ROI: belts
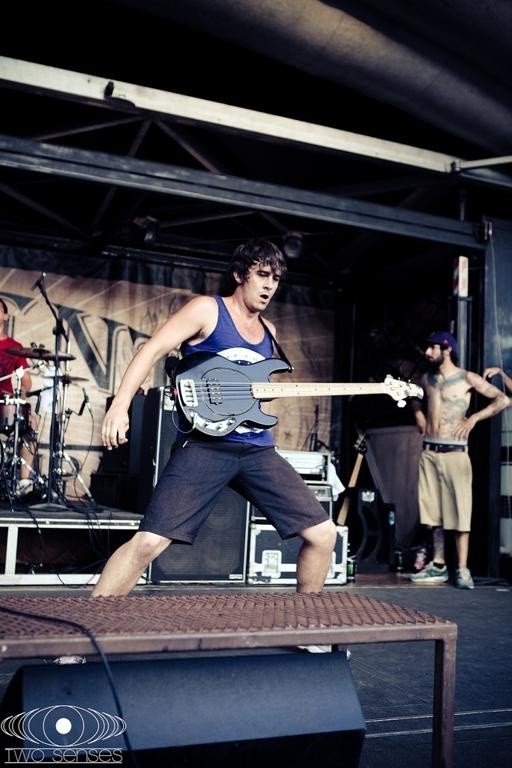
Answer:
[421,440,463,452]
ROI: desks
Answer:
[1,591,458,767]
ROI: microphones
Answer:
[31,272,47,292]
[26,387,52,397]
[310,405,318,451]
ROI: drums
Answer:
[0,397,32,436]
[0,440,20,501]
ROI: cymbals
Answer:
[44,375,89,382]
[4,347,77,361]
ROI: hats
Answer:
[415,330,459,356]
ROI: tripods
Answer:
[9,285,85,514]
[0,404,53,503]
[43,382,97,507]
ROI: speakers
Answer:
[0,652,366,768]
[142,387,251,586]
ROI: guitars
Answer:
[173,351,425,438]
[333,432,370,557]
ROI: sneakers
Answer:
[455,567,475,589]
[409,560,448,583]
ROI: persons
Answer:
[0,299,38,499]
[54,237,351,664]
[482,367,512,393]
[409,330,510,590]
